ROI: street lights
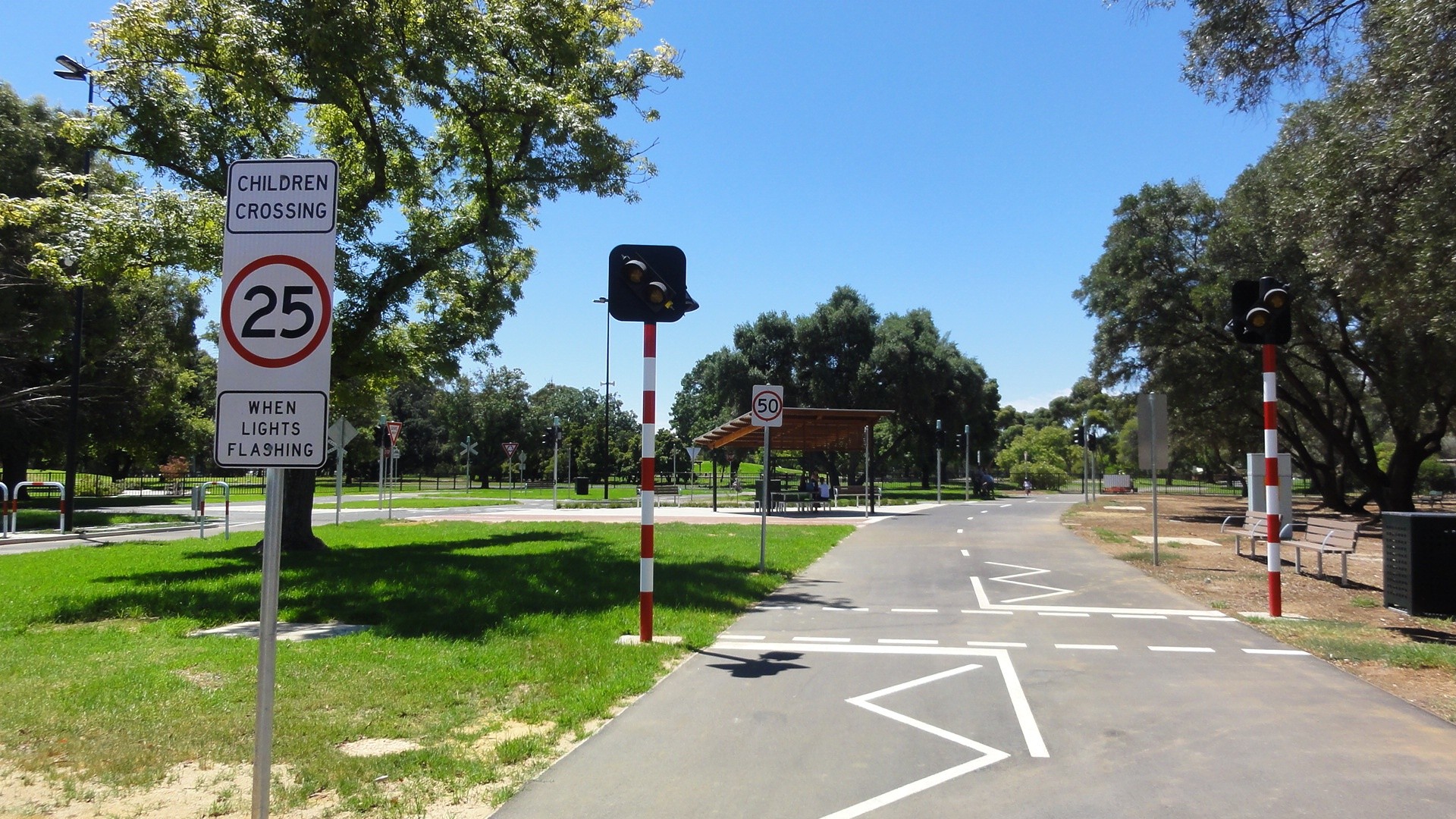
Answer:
[52,53,116,532]
[592,297,609,499]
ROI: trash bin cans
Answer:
[1382,511,1456,620]
[754,480,782,508]
[573,477,589,495]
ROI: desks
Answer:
[770,492,820,513]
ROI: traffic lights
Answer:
[955,432,966,456]
[372,424,383,448]
[608,244,687,323]
[1073,424,1085,447]
[1232,278,1292,345]
[542,425,555,448]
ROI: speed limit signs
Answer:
[751,384,783,427]
[220,254,331,367]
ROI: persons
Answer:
[761,474,764,479]
[1118,470,1126,475]
[971,463,996,501]
[733,479,742,492]
[1024,477,1031,495]
[798,467,829,511]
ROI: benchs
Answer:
[1221,511,1293,561]
[754,499,809,513]
[637,486,682,507]
[833,486,881,508]
[779,501,831,514]
[1417,490,1444,509]
[1280,518,1359,585]
[525,480,556,495]
